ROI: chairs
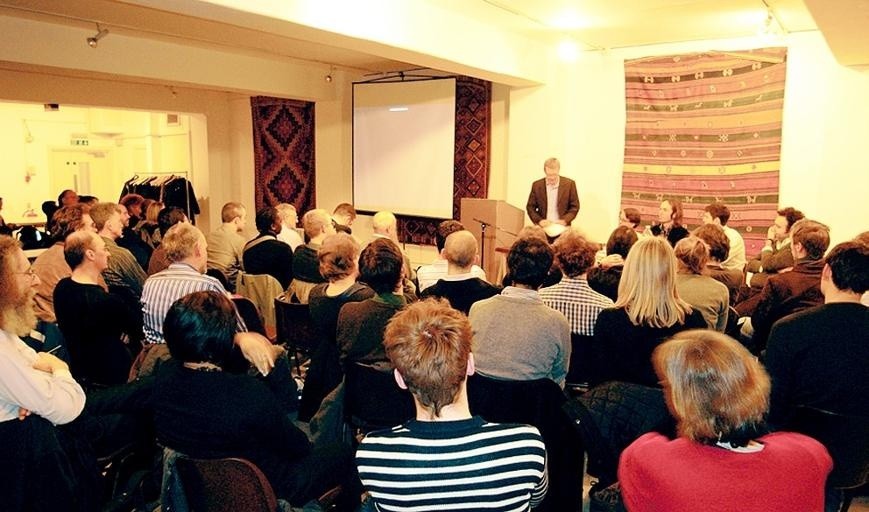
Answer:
[1,266,869,511]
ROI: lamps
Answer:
[84,19,109,48]
[322,64,339,86]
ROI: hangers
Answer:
[127,173,183,185]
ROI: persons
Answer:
[418,198,869,410]
[354,297,549,512]
[617,330,832,511]
[526,159,580,240]
[0,190,422,511]
